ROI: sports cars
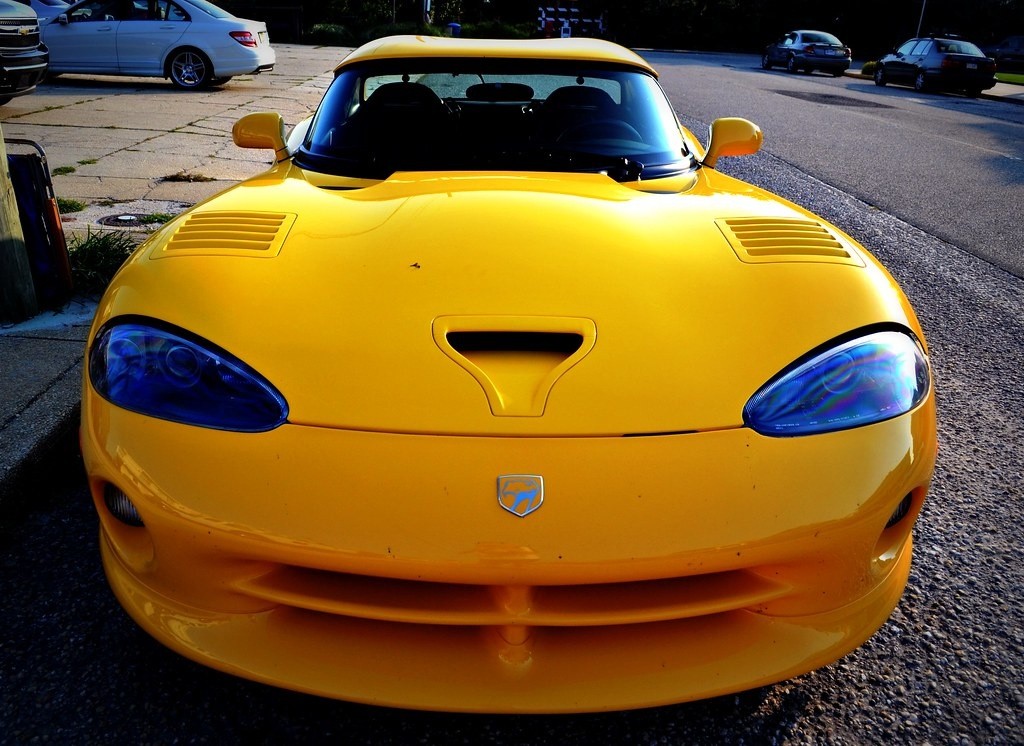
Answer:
[76,36,944,719]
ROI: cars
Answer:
[872,36,997,100]
[37,0,277,90]
[0,1,49,107]
[760,30,854,76]
[15,0,90,16]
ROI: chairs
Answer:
[155,7,166,20]
[346,80,639,172]
